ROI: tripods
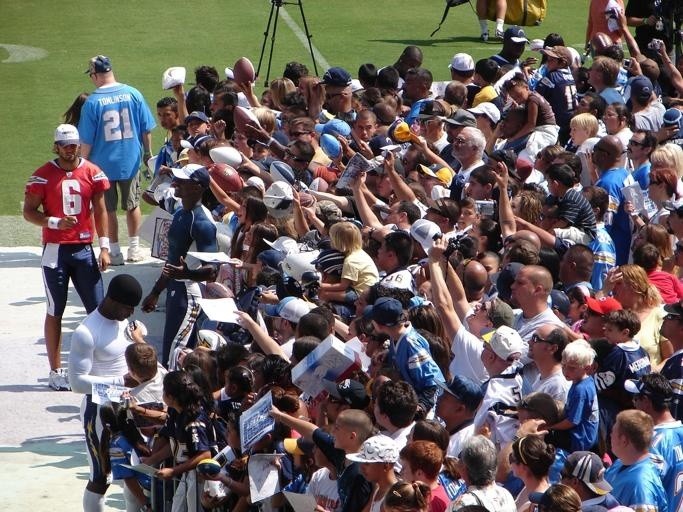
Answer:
[255,0,319,86]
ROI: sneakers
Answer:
[107,252,126,267]
[127,247,148,262]
[48,369,71,392]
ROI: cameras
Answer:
[120,395,130,408]
[623,58,633,68]
[475,200,494,216]
[648,39,663,49]
[433,232,458,256]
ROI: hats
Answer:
[108,274,142,307]
[180,134,214,151]
[262,24,683,272]
[283,435,316,459]
[363,296,403,326]
[183,110,210,127]
[258,296,310,323]
[170,164,210,188]
[54,124,82,149]
[623,379,652,396]
[564,451,615,495]
[319,377,369,410]
[479,325,524,363]
[582,295,623,318]
[83,54,112,74]
[431,375,483,406]
[344,434,401,464]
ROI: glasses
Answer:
[509,452,517,464]
[531,333,552,346]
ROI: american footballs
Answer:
[234,57,256,88]
[297,191,313,208]
[208,164,243,192]
[270,161,295,186]
[309,177,329,193]
[320,133,340,158]
[514,158,535,182]
[281,396,310,420]
[205,281,235,298]
[590,32,613,56]
[210,147,242,169]
[233,105,260,137]
[196,459,222,476]
[161,66,186,89]
